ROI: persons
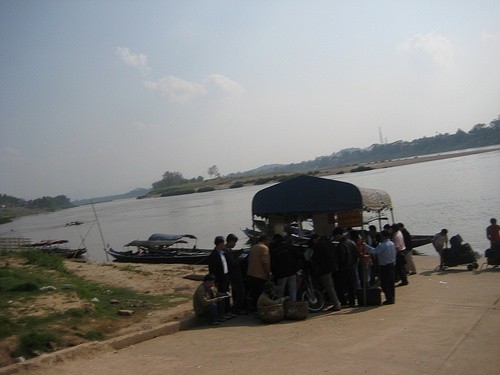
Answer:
[486,218,500,268]
[193,223,417,326]
[432,229,448,271]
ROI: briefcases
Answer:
[356,285,381,306]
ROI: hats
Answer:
[389,224,400,229]
[264,281,277,291]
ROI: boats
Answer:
[242,213,437,250]
[20,239,87,258]
[108,232,243,265]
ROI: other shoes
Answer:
[383,300,394,305]
[209,321,219,324]
[219,316,230,319]
[398,282,408,286]
[227,312,237,317]
[216,318,226,322]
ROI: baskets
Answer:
[284,301,308,320]
[269,304,285,322]
